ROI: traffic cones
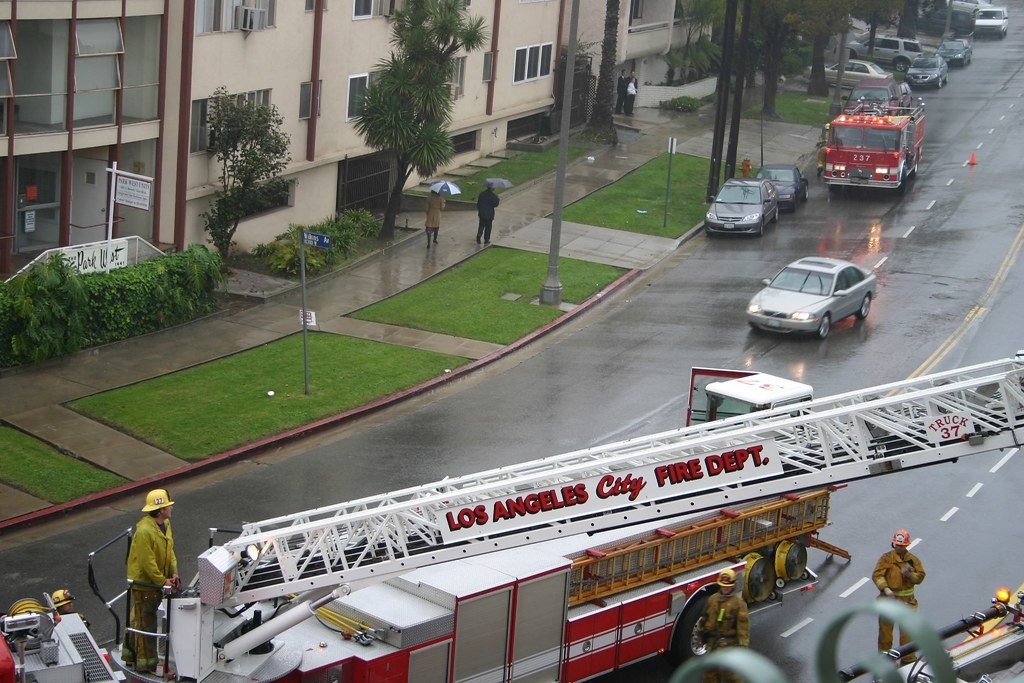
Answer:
[967,152,978,165]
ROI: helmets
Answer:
[51,590,74,607]
[892,529,910,546]
[717,569,736,587]
[141,486,175,512]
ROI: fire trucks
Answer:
[0,350,1024,683]
[822,97,925,195]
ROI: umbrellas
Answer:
[480,178,514,188]
[427,180,462,195]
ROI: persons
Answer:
[624,77,638,116]
[872,529,926,655]
[615,69,629,114]
[52,589,89,628]
[121,489,180,674]
[626,71,638,114]
[691,569,751,683]
[424,190,445,248]
[476,187,500,244]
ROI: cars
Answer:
[756,163,809,213]
[704,177,779,238]
[946,0,995,15]
[903,53,949,89]
[804,59,893,88]
[897,80,913,113]
[935,38,973,68]
[746,257,877,341]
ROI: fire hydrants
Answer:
[739,159,753,178]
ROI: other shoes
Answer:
[149,669,157,676]
[127,661,135,666]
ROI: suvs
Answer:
[841,78,902,117]
[847,34,923,72]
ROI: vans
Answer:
[919,7,975,36]
[972,7,1009,39]
